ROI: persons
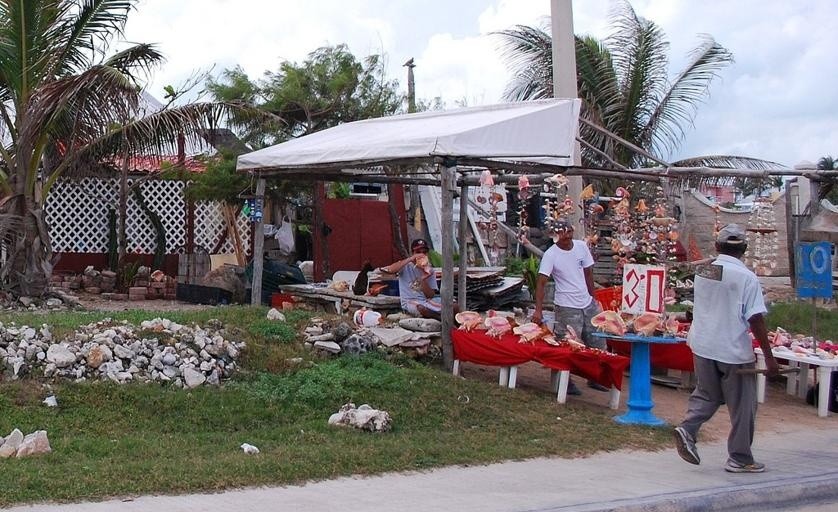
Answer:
[382,238,461,325]
[675,225,778,473]
[531,222,610,396]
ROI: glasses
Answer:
[416,251,427,253]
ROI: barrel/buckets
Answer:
[272,292,294,309]
[354,310,385,328]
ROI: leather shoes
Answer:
[587,380,609,392]
[567,375,581,395]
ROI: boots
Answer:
[673,426,700,465]
[724,456,766,473]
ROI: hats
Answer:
[411,239,430,250]
[718,223,746,244]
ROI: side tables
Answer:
[593,333,689,424]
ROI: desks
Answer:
[453,330,628,411]
[604,321,838,418]
